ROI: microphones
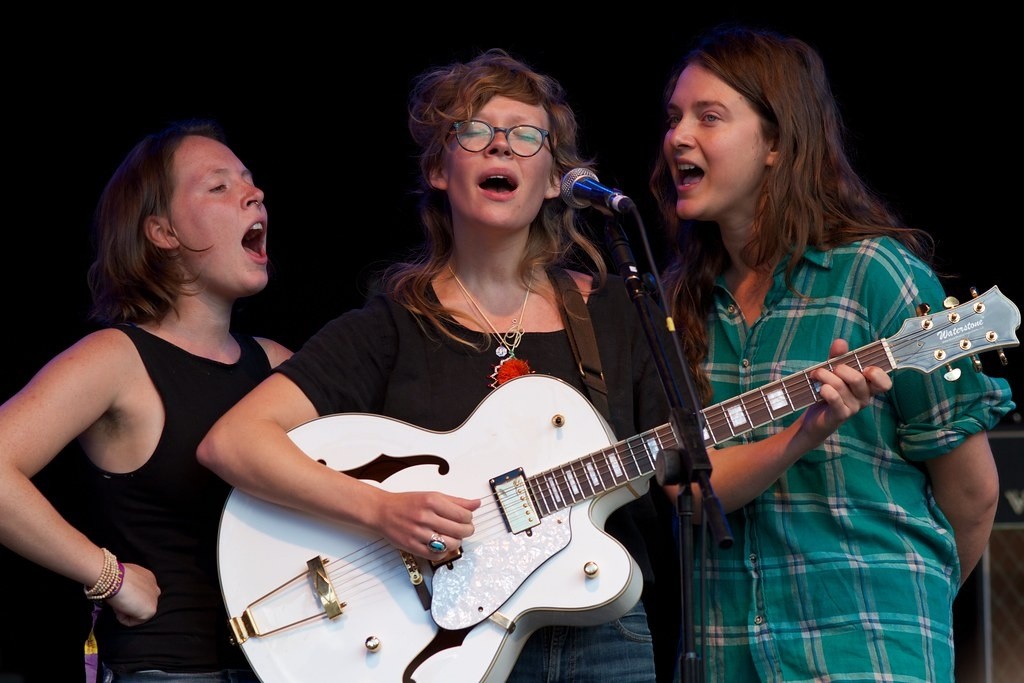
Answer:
[561,167,635,213]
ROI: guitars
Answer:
[219,285,1021,679]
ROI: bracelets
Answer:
[84,547,124,600]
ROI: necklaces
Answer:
[442,251,537,391]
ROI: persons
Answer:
[636,31,1014,683]
[0,120,301,683]
[196,52,892,683]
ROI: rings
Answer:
[427,533,447,553]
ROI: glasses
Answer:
[441,120,563,169]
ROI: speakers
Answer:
[950,430,1024,683]
